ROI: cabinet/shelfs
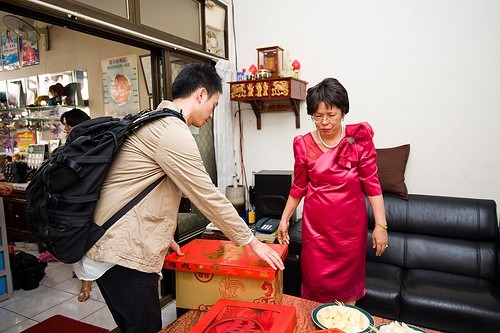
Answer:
[0,101,79,154]
[0,181,38,243]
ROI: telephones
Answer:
[255,217,281,234]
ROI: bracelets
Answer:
[376,223,388,230]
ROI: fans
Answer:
[2,14,49,52]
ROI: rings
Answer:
[385,245,388,248]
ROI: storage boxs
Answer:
[162,240,297,333]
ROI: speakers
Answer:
[255,169,297,226]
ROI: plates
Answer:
[369,323,427,333]
[311,302,374,333]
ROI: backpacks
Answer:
[26,109,186,264]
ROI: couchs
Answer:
[283,194,500,333]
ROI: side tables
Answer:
[253,168,295,225]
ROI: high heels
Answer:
[78,280,92,302]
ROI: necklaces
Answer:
[318,127,344,149]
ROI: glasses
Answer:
[311,114,341,123]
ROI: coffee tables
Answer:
[157,293,442,333]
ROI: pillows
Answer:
[376,143,410,200]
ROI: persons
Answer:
[71,63,285,333]
[5,30,14,48]
[60,109,92,302]
[0,182,14,194]
[5,155,12,164]
[277,78,388,308]
[48,83,70,105]
